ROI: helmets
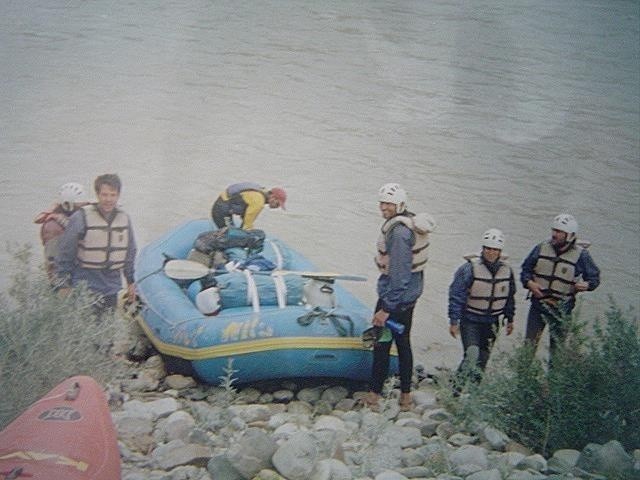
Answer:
[117,290,147,324]
[56,182,87,211]
[552,214,578,243]
[377,182,409,215]
[481,229,505,252]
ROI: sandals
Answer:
[353,392,380,405]
[398,398,412,411]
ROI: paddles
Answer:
[165,259,366,280]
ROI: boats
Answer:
[1,373,121,476]
[132,214,400,386]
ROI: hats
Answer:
[271,187,287,211]
[362,326,392,348]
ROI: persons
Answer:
[40,182,88,291]
[520,214,600,373]
[212,181,286,230]
[53,174,137,356]
[353,182,429,412]
[448,228,515,398]
[187,229,265,270]
